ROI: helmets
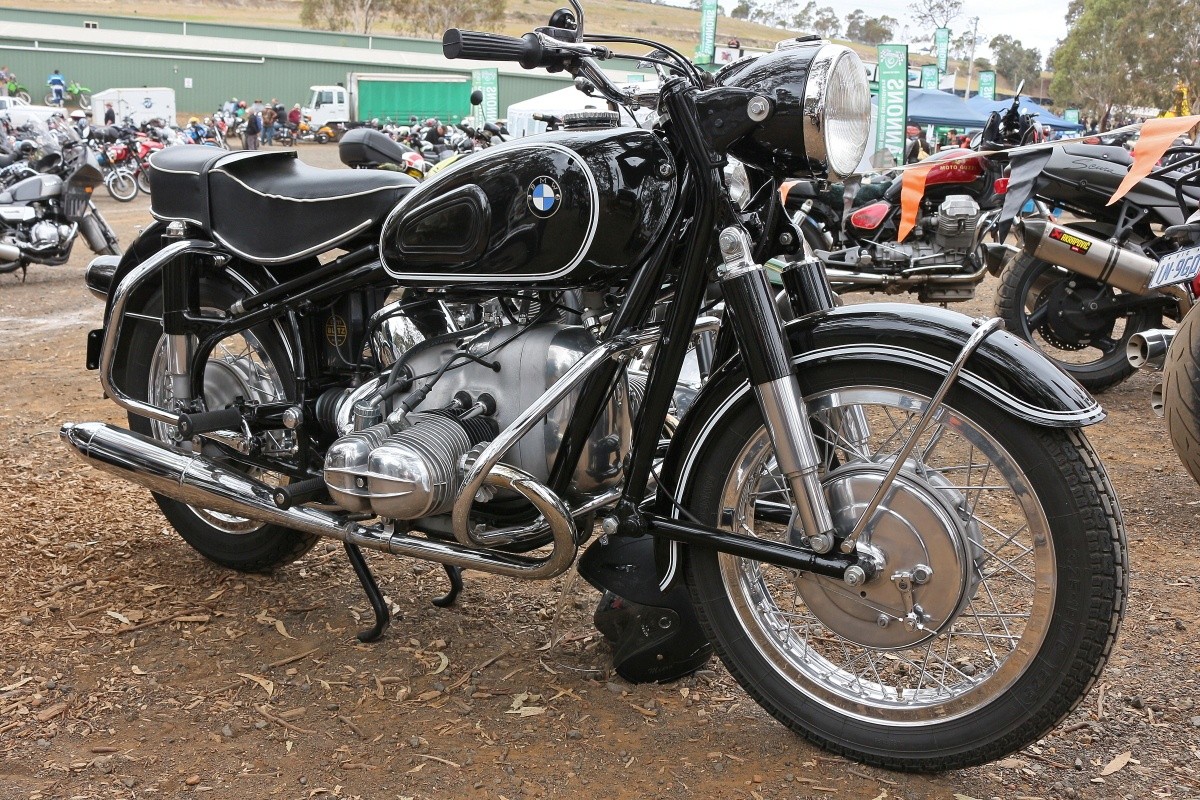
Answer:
[576,530,714,685]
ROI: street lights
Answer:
[964,16,979,101]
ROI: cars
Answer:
[0,96,68,127]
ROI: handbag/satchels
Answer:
[263,110,272,123]
[255,115,262,131]
[917,139,930,160]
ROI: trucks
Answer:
[295,70,474,132]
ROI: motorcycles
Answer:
[4,74,33,104]
[0,87,1200,492]
[43,77,92,110]
[56,0,1131,775]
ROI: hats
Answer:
[265,104,272,108]
[495,122,504,125]
[294,103,301,109]
[441,124,448,131]
[190,117,197,122]
[239,101,246,107]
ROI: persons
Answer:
[0,67,10,96]
[288,104,302,124]
[105,102,115,125]
[230,97,287,150]
[496,122,509,135]
[947,130,961,148]
[906,122,932,164]
[1080,113,1125,137]
[425,125,449,144]
[47,69,65,108]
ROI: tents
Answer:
[1106,103,1162,117]
[508,84,653,139]
[872,85,1084,154]
